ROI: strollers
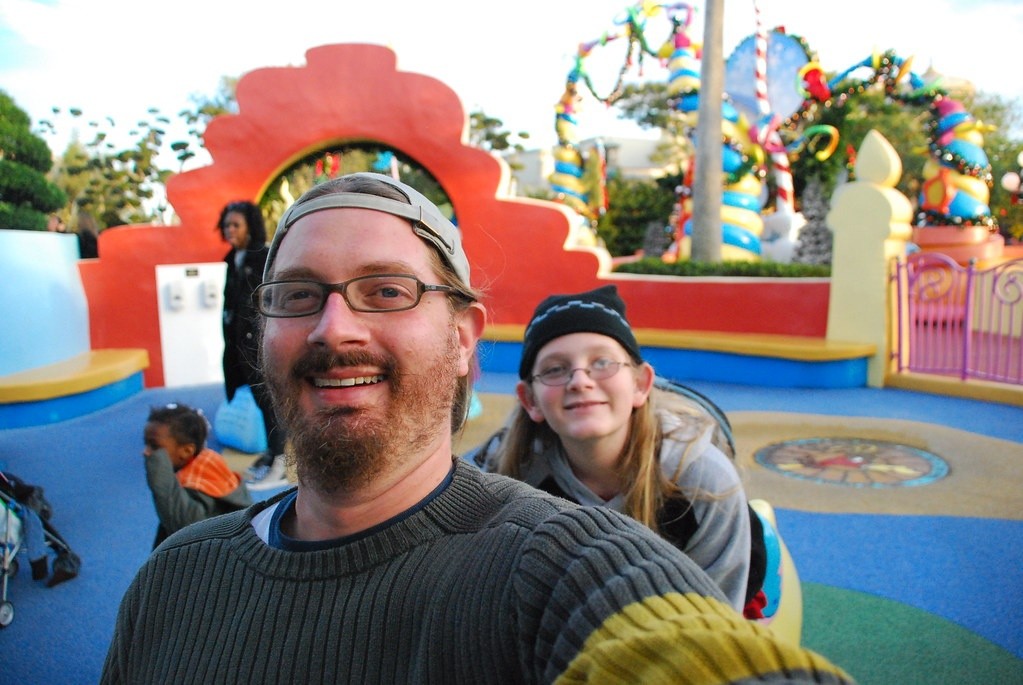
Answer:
[1,469,82,626]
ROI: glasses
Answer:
[528,355,631,385]
[252,274,454,319]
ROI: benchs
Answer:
[1,350,149,429]
[474,323,876,390]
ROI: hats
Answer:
[256,171,469,291]
[518,284,642,379]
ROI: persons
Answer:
[216,201,293,489]
[101,170,865,684]
[142,401,255,555]
[476,285,755,624]
